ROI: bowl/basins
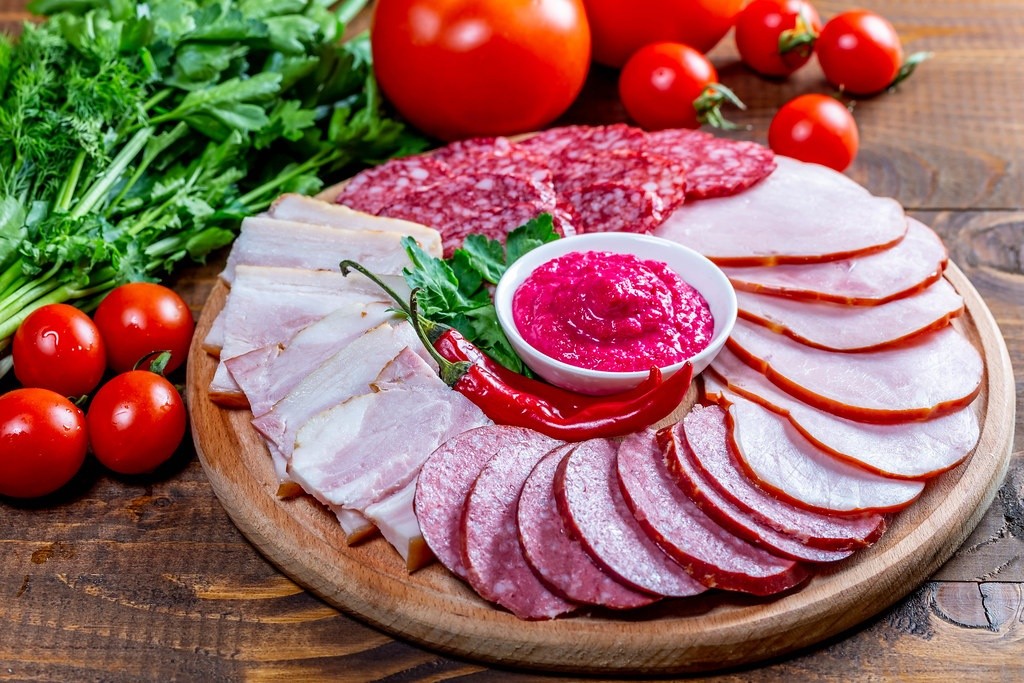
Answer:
[494,231,738,394]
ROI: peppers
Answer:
[339,260,692,442]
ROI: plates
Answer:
[186,131,1017,669]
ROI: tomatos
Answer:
[0,281,192,497]
[368,0,933,174]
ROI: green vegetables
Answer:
[0,0,427,349]
[395,212,566,378]
